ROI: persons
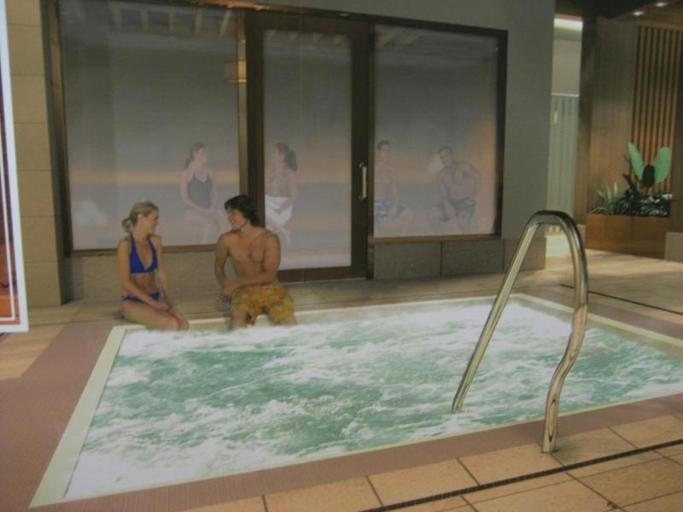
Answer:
[116,199,190,331]
[264,142,300,249]
[426,145,481,233]
[213,194,298,331]
[181,142,220,242]
[374,139,413,237]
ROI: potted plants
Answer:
[583,181,671,257]
